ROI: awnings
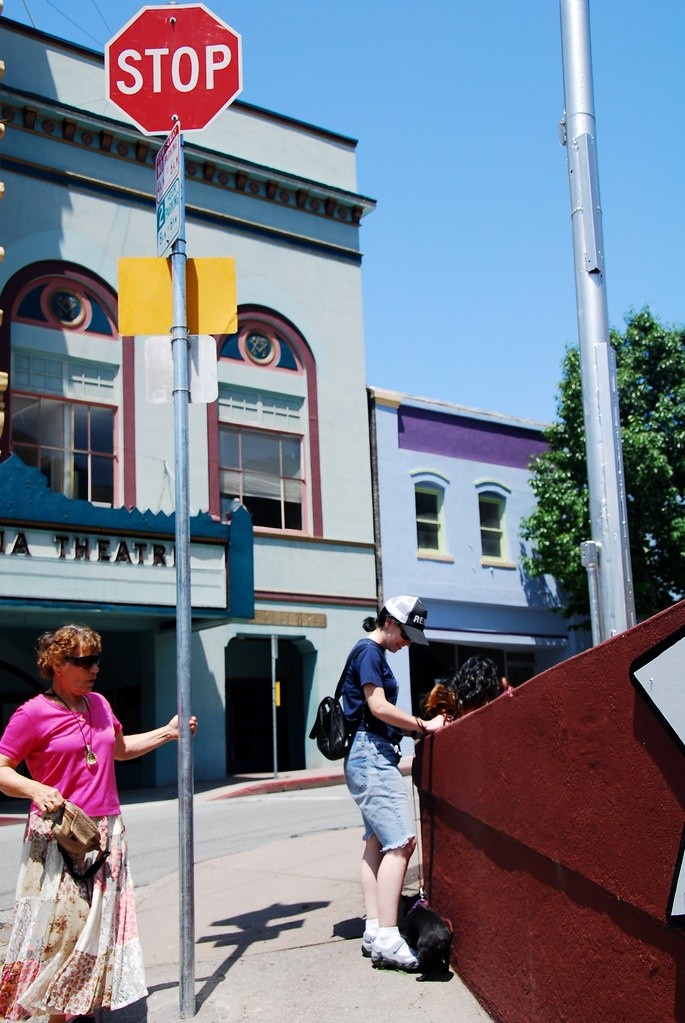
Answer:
[422,629,568,653]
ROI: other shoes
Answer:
[361,931,376,957]
[371,938,420,973]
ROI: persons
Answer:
[420,656,513,724]
[343,597,445,971]
[0,623,196,1023]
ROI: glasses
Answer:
[65,655,102,669]
[396,624,411,640]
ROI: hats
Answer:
[384,595,431,646]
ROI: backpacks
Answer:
[309,642,379,761]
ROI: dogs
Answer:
[397,891,454,982]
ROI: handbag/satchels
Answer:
[50,799,112,881]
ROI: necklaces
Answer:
[51,686,97,765]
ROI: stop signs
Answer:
[105,3,244,136]
